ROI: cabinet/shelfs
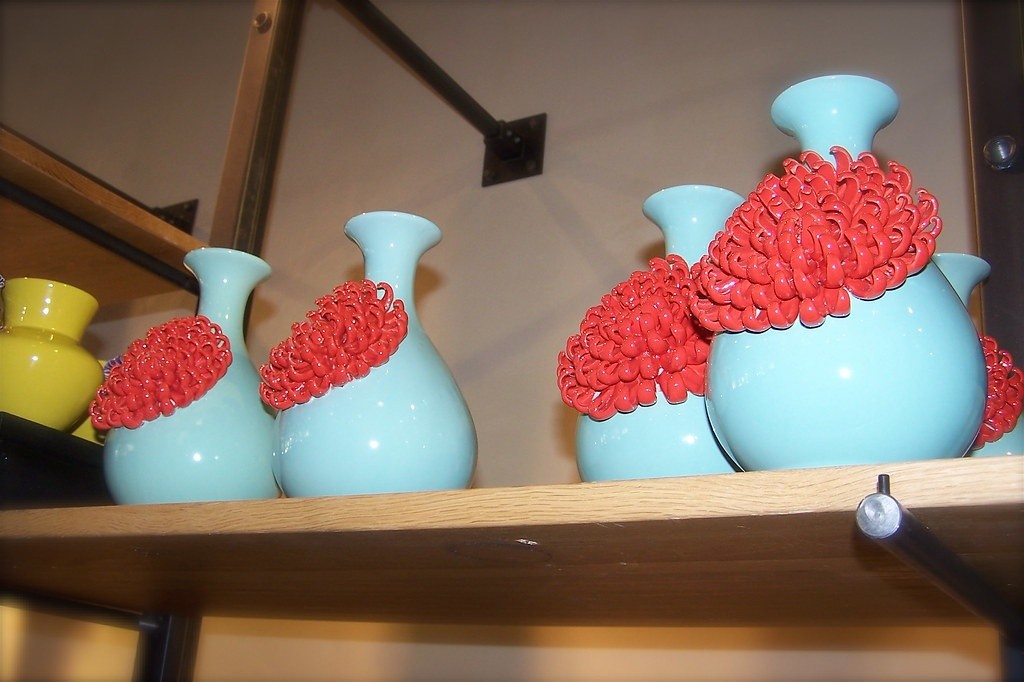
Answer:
[1,0,1024,682]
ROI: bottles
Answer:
[558,184,746,482]
[0,277,102,432]
[690,76,986,470]
[930,253,1023,460]
[260,210,476,496]
[93,245,278,498]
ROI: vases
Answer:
[268,211,479,496]
[0,276,105,430]
[928,250,1024,458]
[704,73,988,471]
[576,185,736,480]
[104,247,282,505]
